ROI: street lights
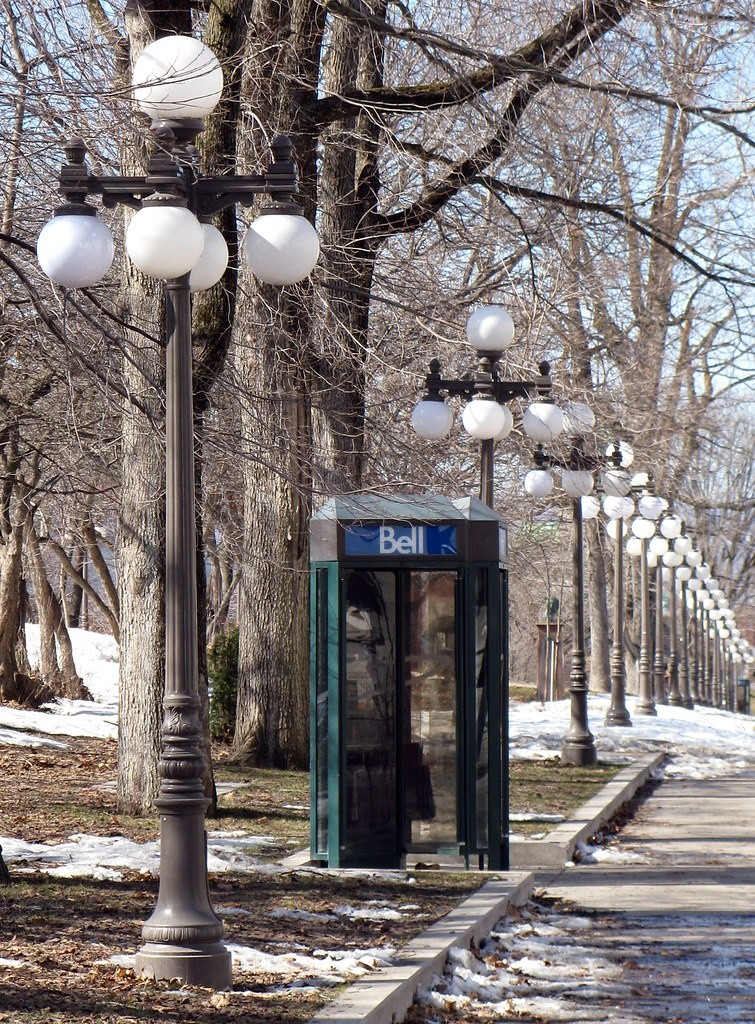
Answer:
[36,33,319,991]
[625,496,755,711]
[582,439,661,727]
[413,306,564,848]
[524,402,632,767]
[607,473,681,717]
